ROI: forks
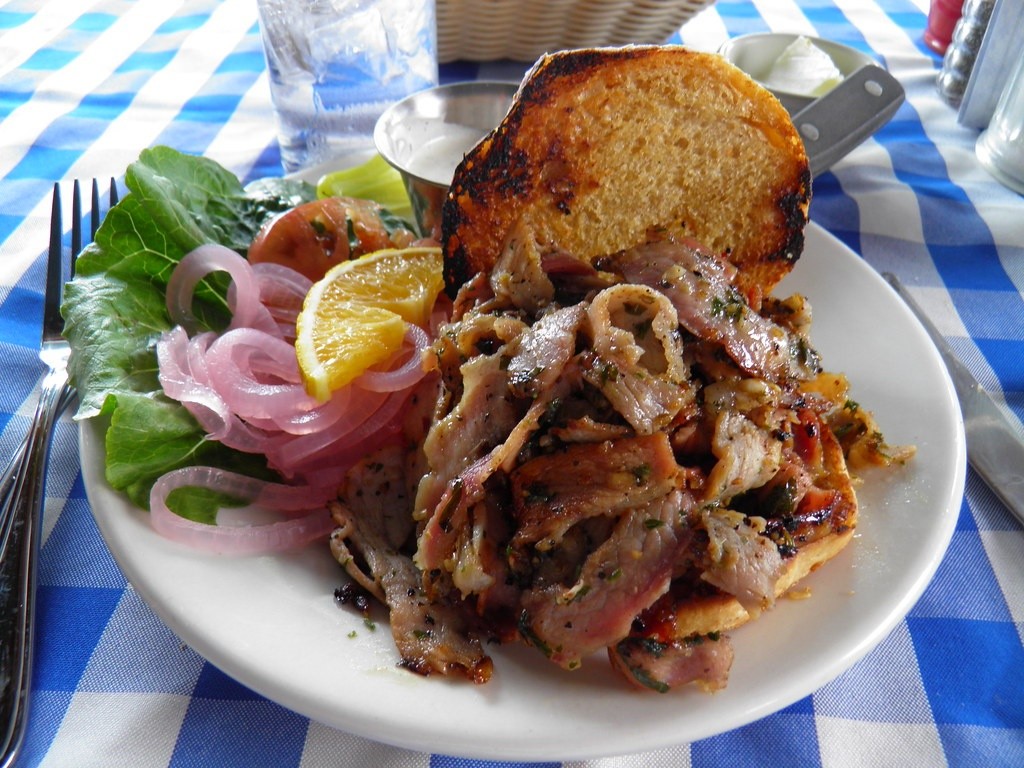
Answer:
[0,179,117,767]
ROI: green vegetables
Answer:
[60,144,421,524]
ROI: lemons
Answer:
[295,247,443,402]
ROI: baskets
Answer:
[257,1,715,66]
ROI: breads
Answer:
[442,45,810,300]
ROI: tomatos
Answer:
[245,198,399,284]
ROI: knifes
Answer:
[882,271,1024,527]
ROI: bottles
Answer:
[924,0,1024,197]
[256,0,440,174]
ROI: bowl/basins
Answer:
[374,81,522,238]
[715,31,886,116]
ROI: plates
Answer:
[80,195,967,761]
